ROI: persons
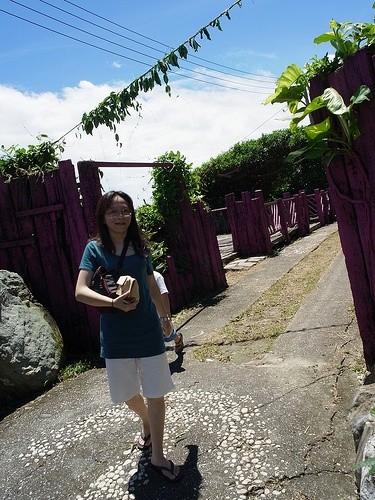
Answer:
[152,270,183,354]
[74,191,184,483]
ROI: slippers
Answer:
[138,429,151,449]
[148,460,184,483]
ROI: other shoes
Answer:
[174,332,183,354]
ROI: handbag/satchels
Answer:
[89,237,140,315]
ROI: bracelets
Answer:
[112,299,113,308]
[160,317,171,322]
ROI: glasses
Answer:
[105,208,133,219]
